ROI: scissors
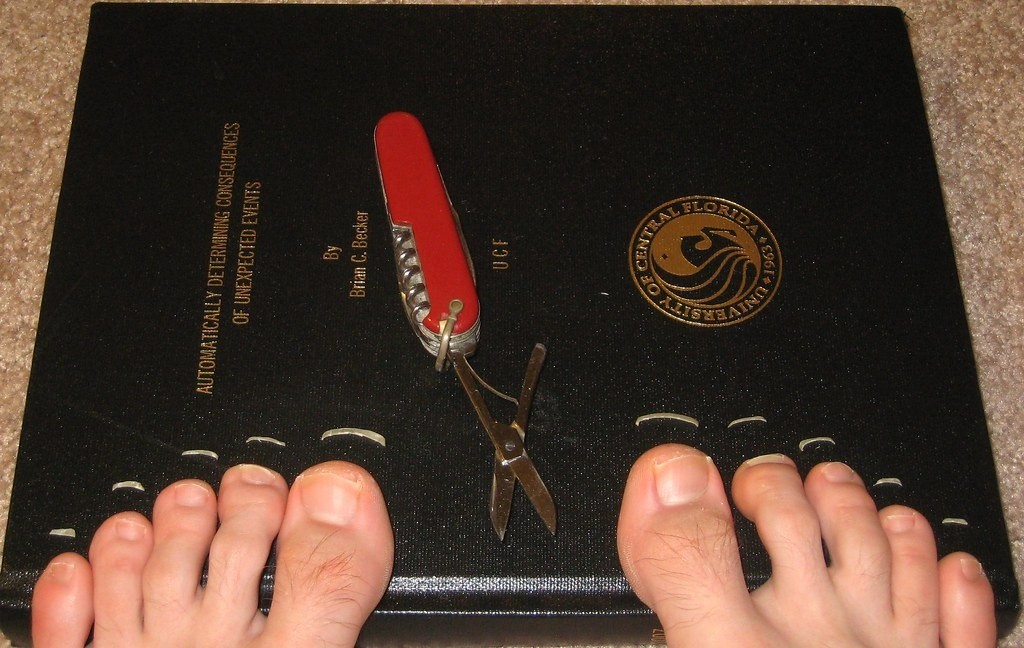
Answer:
[449,342,557,541]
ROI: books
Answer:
[1,4,1020,648]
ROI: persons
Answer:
[33,442,998,648]
